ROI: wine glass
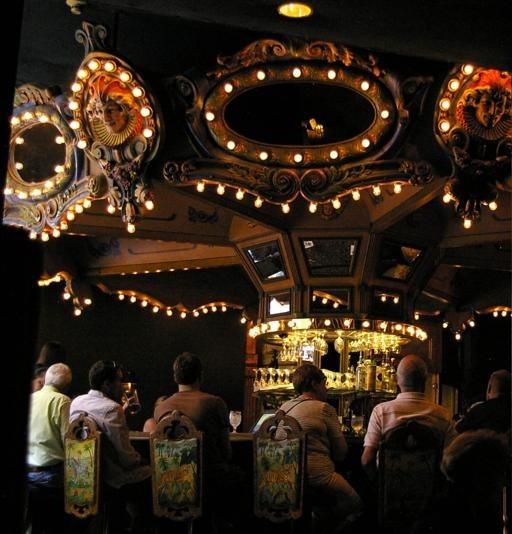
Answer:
[229,410,240,432]
[351,416,364,433]
[250,336,304,392]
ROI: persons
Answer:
[452,413,461,427]
[463,66,512,126]
[68,357,153,493]
[85,72,141,132]
[456,368,511,434]
[153,351,235,482]
[26,360,76,496]
[360,353,457,473]
[31,366,49,393]
[274,364,363,532]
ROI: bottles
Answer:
[352,347,398,395]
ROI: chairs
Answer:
[57,408,105,534]
[373,412,452,534]
[147,410,216,533]
[243,410,313,532]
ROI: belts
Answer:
[26,461,63,473]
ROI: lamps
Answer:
[274,3,316,20]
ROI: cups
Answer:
[125,389,146,414]
